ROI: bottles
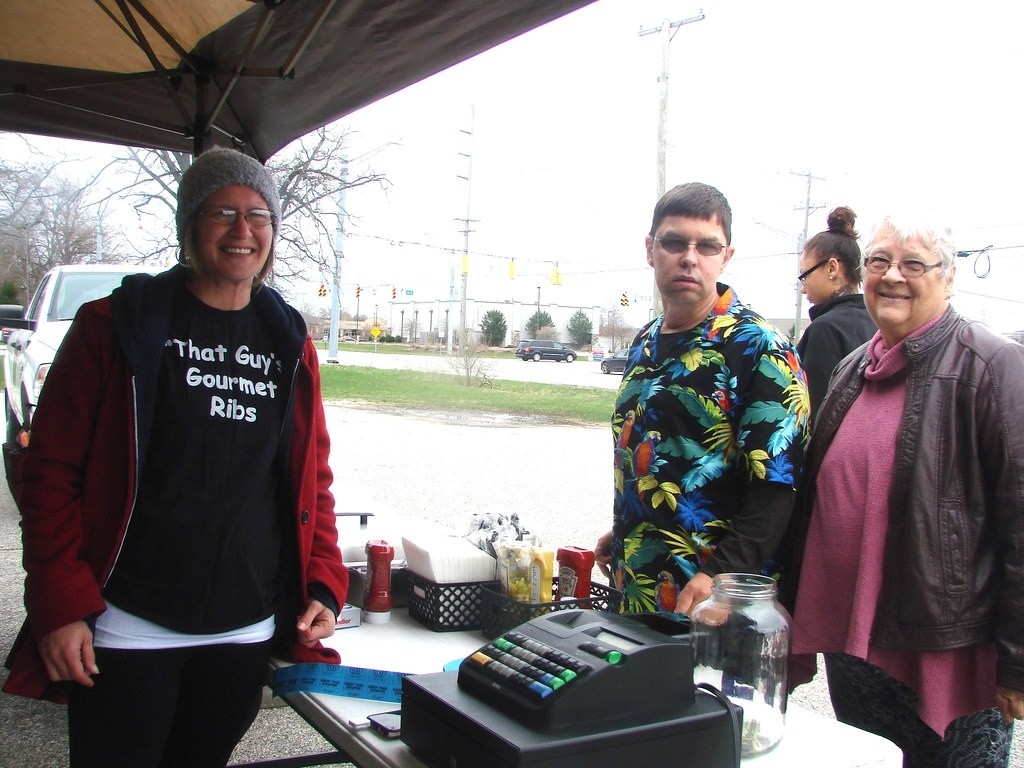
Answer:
[688,572,792,757]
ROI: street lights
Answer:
[537,286,541,329]
[375,305,378,326]
[649,309,652,322]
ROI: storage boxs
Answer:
[400,569,502,632]
[478,576,624,638]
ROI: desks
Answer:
[221,606,902,768]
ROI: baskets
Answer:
[478,577,623,641]
[399,569,501,633]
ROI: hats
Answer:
[942,707,1014,768]
[176,144,282,241]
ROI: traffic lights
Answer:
[621,292,629,306]
[318,284,327,297]
[392,288,396,299]
[356,286,360,297]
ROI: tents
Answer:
[1,1,591,165]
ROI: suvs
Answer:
[0,264,166,438]
[516,340,577,363]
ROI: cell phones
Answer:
[366,709,401,737]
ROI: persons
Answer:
[795,205,878,425]
[2,148,437,768]
[595,182,811,703]
[765,204,1024,768]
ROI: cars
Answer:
[600,349,629,374]
[1,326,18,343]
[341,336,354,341]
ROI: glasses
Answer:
[798,258,841,286]
[864,257,942,279]
[197,205,277,227]
[653,235,727,256]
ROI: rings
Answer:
[704,617,715,626]
